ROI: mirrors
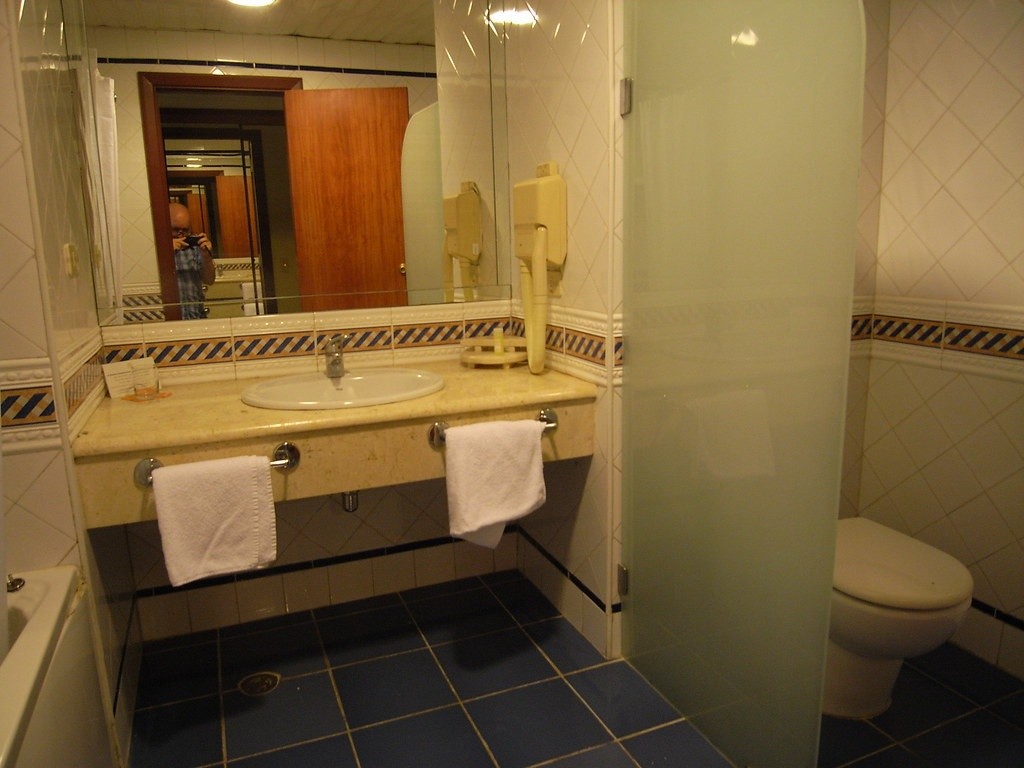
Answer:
[60,1,513,327]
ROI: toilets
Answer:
[822,515,977,723]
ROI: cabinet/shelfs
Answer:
[202,283,245,318]
[8,585,116,768]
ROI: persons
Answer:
[169,203,215,320]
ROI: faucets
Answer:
[321,332,349,382]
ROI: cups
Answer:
[130,363,159,401]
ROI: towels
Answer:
[150,454,280,589]
[241,281,264,316]
[439,418,550,553]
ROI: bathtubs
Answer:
[0,561,108,768]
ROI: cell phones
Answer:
[184,236,204,247]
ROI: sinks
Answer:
[237,363,445,412]
